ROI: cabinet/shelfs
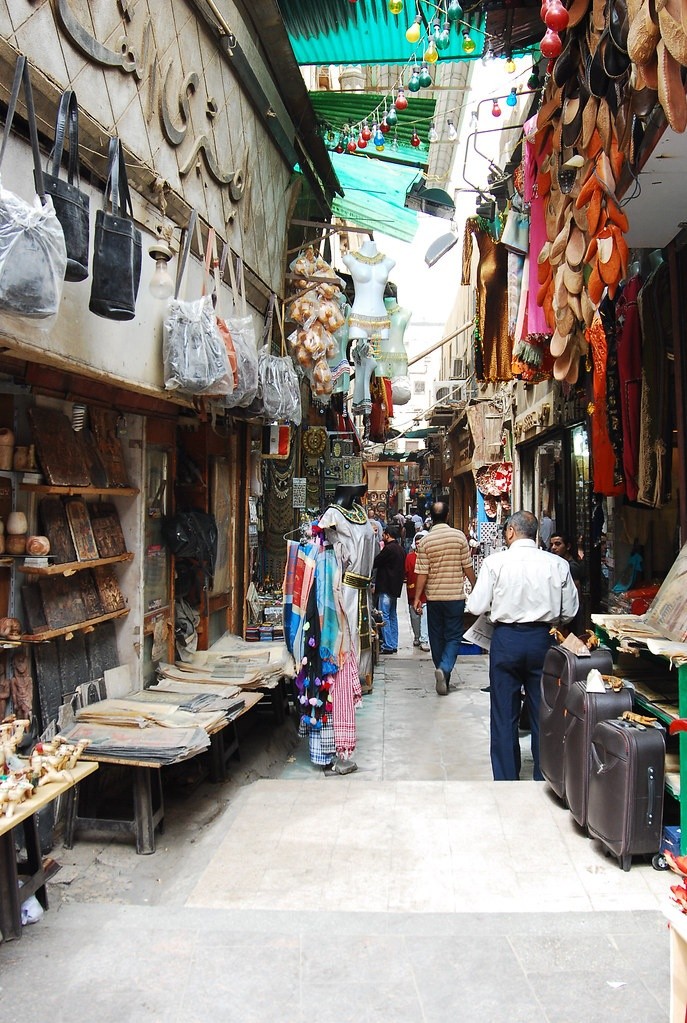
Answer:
[592,615,687,870]
[0,394,141,649]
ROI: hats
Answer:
[405,515,412,519]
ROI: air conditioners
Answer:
[450,356,466,380]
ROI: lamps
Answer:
[324,0,568,154]
[462,124,525,222]
[326,144,457,222]
[147,178,175,299]
[424,187,491,268]
[473,88,544,200]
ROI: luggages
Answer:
[564,669,635,828]
[539,632,613,808]
[586,712,670,871]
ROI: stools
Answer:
[62,675,299,855]
[0,812,50,944]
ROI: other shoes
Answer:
[379,647,393,655]
[420,642,431,651]
[525,0,687,386]
[393,649,397,653]
[435,668,450,695]
[414,637,422,646]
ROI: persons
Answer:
[374,297,413,377]
[342,241,397,339]
[319,483,376,694]
[373,508,405,654]
[465,511,584,780]
[413,501,476,696]
[378,509,434,652]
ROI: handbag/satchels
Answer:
[164,442,218,593]
[89,138,141,319]
[162,209,303,438]
[33,89,89,282]
[0,56,68,330]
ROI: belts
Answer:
[494,621,551,628]
